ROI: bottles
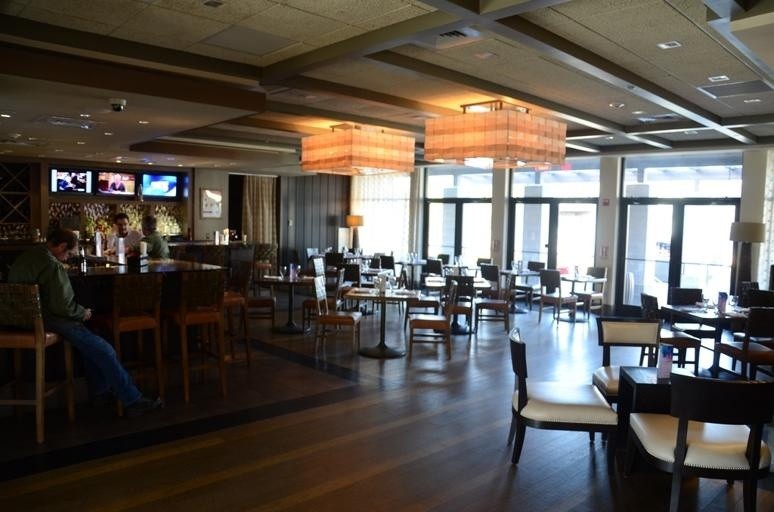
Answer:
[32,229,41,244]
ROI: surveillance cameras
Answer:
[110,98,126,112]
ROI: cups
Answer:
[80,258,88,275]
[511,261,518,272]
[729,295,739,307]
[322,247,370,274]
[408,252,465,267]
[518,260,523,273]
[378,273,387,294]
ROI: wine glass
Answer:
[388,276,397,294]
[702,291,711,314]
[374,278,382,296]
[712,295,718,314]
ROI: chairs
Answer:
[1,249,278,444]
[506,283,774,510]
[232,248,609,364]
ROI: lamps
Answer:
[346,215,364,252]
[424,100,567,167]
[299,123,416,176]
[731,222,764,292]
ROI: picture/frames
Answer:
[199,186,224,220]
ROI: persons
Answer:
[110,214,171,260]
[4,228,165,419]
[107,173,128,193]
[103,210,141,256]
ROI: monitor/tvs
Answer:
[48,167,95,196]
[140,169,183,202]
[95,168,140,201]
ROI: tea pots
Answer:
[279,260,304,282]
